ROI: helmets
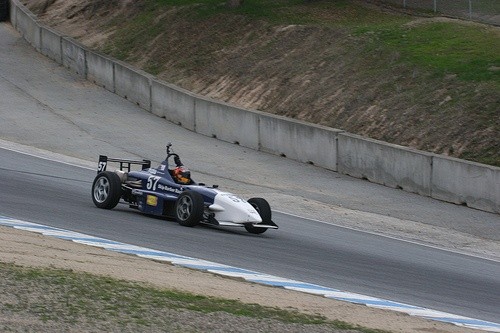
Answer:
[173,166,191,184]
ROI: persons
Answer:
[172,166,191,184]
